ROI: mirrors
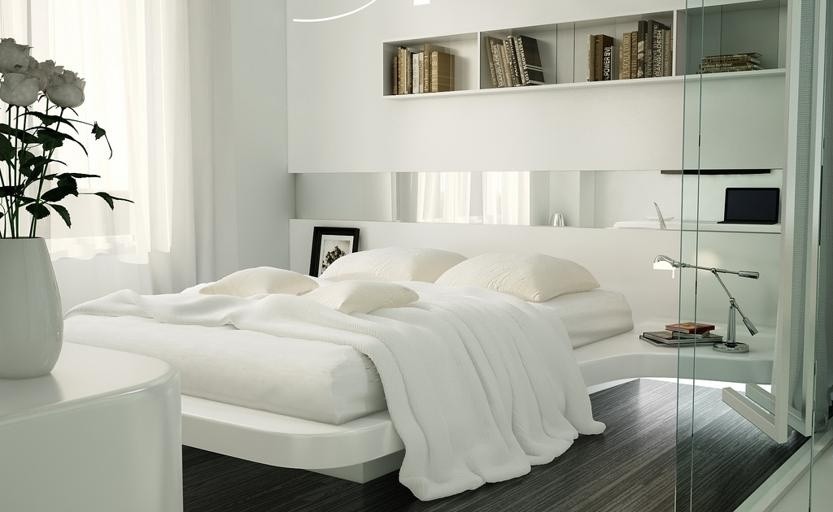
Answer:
[395,169,784,234]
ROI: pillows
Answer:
[298,281,418,315]
[433,253,598,302]
[200,266,318,298]
[318,248,466,285]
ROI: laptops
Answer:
[716,187,781,225]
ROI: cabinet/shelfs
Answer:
[380,1,789,102]
[1,342,182,512]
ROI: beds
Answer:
[61,220,785,483]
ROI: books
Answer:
[392,46,454,94]
[485,33,546,87]
[697,53,765,74]
[639,321,724,348]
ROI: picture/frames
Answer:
[309,227,360,277]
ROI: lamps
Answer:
[653,256,760,353]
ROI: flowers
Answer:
[1,37,135,237]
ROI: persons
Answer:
[588,19,671,81]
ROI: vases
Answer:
[0,238,63,379]
